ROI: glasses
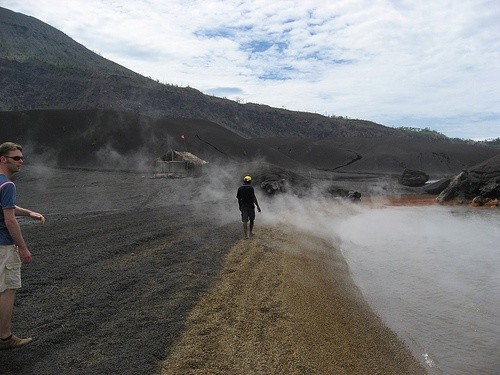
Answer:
[4,155,23,161]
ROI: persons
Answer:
[0,141,45,349]
[236,175,261,239]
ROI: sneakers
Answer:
[0,334,32,349]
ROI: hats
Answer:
[244,176,251,181]
